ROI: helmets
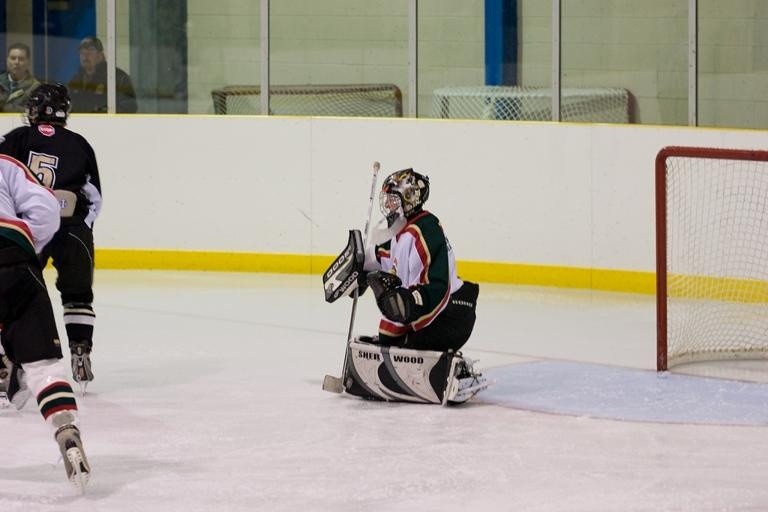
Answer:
[26,83,71,126]
[378,168,430,219]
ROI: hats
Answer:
[79,38,103,51]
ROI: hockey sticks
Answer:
[322,162,380,393]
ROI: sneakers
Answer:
[54,424,90,480]
[0,354,26,402]
[71,344,93,381]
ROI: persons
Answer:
[1,134,91,484]
[322,168,488,405]
[0,42,43,113]
[67,35,137,113]
[0,80,103,393]
